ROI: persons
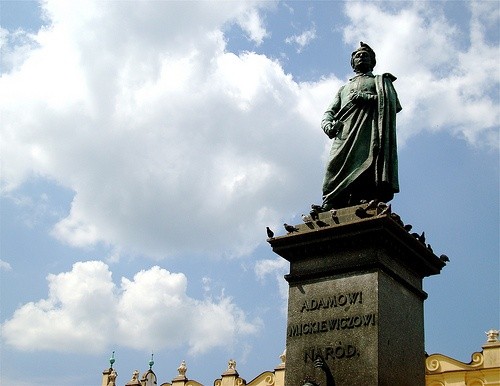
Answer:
[320,41,402,203]
